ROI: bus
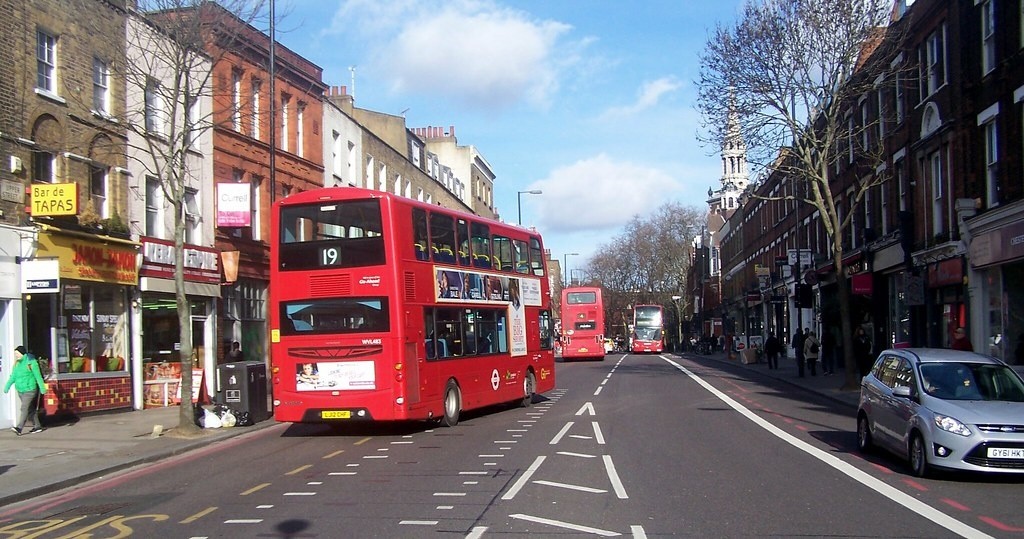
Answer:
[633,304,665,355]
[561,286,606,361]
[627,324,635,351]
[267,188,554,428]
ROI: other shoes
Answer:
[829,372,834,376]
[823,371,828,376]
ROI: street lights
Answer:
[563,252,580,288]
[518,190,542,225]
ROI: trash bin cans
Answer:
[217,361,269,425]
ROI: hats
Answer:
[14,345,26,355]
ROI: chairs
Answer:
[414,241,526,268]
[427,330,491,360]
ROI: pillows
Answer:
[49,356,125,373]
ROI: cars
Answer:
[604,337,629,355]
[856,347,1024,478]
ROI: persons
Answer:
[439,272,451,297]
[688,333,719,355]
[666,332,686,354]
[226,342,244,363]
[723,332,738,353]
[821,327,834,376]
[4,346,46,435]
[719,332,726,352]
[618,339,623,350]
[952,328,973,351]
[447,334,460,353]
[765,332,779,370]
[853,329,871,381]
[792,328,820,377]
[483,275,492,300]
[461,274,472,299]
[554,329,560,353]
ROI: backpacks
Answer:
[807,338,819,353]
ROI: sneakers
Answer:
[29,427,42,434]
[11,427,22,436]
